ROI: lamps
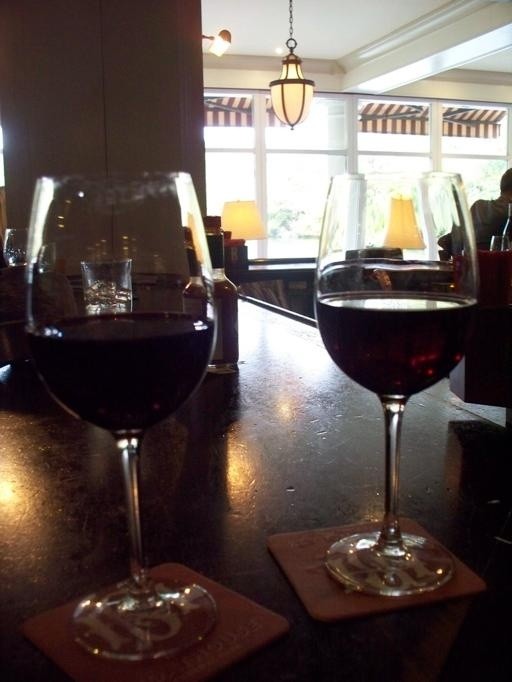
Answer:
[202,29,233,59]
[267,0,316,133]
[220,198,270,241]
[382,196,427,250]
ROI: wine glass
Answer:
[313,173,481,598]
[23,168,221,665]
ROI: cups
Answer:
[4,227,58,271]
[78,259,133,313]
[488,234,509,250]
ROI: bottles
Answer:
[502,202,512,252]
[184,215,239,375]
[202,216,248,274]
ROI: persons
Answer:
[470,167,512,243]
